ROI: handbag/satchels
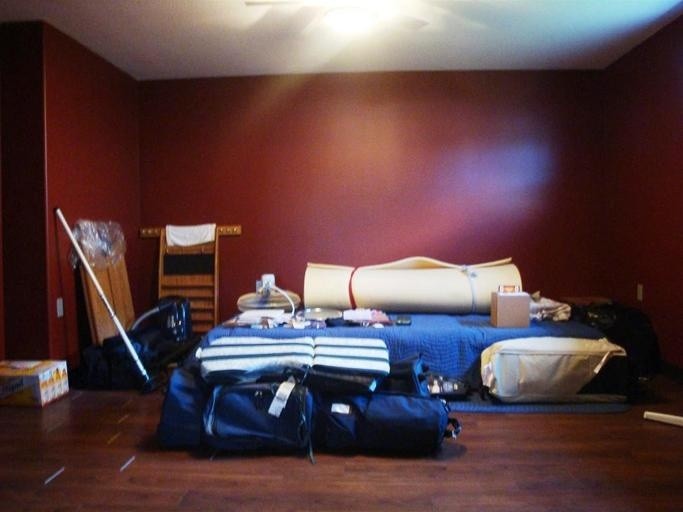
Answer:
[190,352,463,459]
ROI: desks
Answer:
[0,359,68,409]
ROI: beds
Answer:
[207,311,624,407]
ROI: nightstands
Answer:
[236,290,301,313]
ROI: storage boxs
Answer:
[489,289,530,328]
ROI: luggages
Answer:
[476,334,639,407]
